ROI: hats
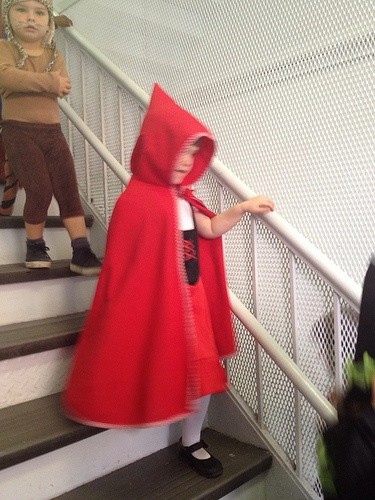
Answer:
[2,0,57,50]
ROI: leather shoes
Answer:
[178,436,224,479]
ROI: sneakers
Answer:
[70,248,103,276]
[24,243,52,268]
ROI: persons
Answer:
[0,0,103,276]
[58,83,274,478]
